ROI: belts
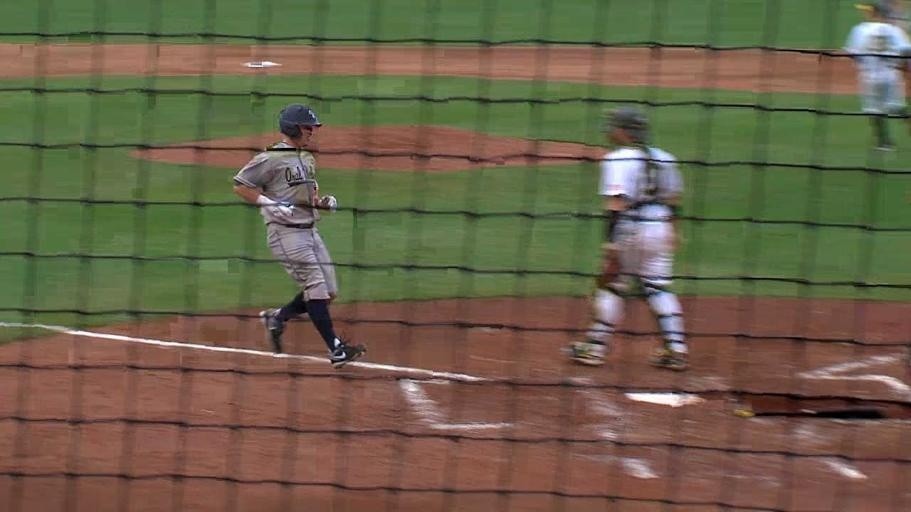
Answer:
[286,224,313,229]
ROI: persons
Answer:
[569,110,694,375]
[842,1,911,150]
[232,103,366,368]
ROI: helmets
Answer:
[279,103,321,137]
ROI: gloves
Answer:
[272,202,295,217]
[319,196,337,211]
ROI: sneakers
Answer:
[646,347,688,371]
[569,343,606,366]
[329,345,365,368]
[259,308,285,353]
